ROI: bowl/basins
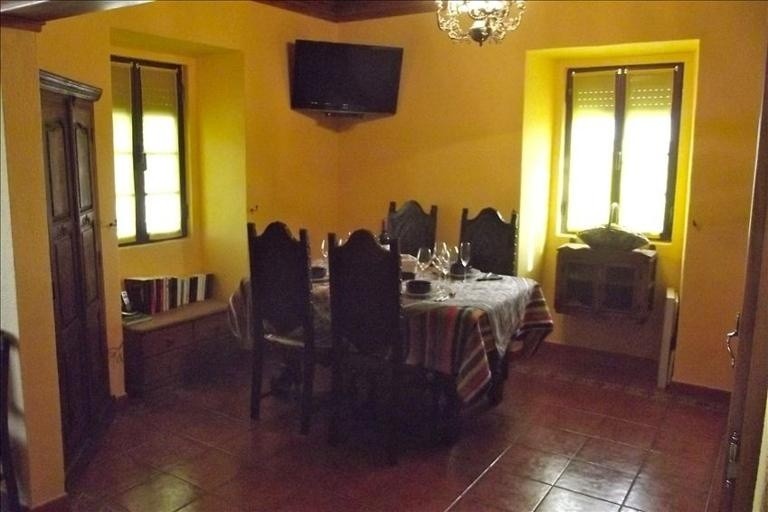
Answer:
[404,280,432,294]
[310,266,328,278]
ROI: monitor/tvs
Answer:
[292,39,404,115]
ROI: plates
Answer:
[400,277,447,297]
[446,268,483,279]
[309,275,330,283]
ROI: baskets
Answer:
[579,204,649,250]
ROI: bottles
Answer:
[380,217,394,251]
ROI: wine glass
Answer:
[417,239,472,289]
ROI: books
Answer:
[119,272,214,327]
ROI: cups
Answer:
[320,231,352,258]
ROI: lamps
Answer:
[434,0,527,48]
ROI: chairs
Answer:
[247,218,333,430]
[457,203,519,276]
[326,229,460,467]
[380,199,442,263]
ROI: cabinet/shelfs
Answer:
[554,243,657,324]
[122,299,228,402]
